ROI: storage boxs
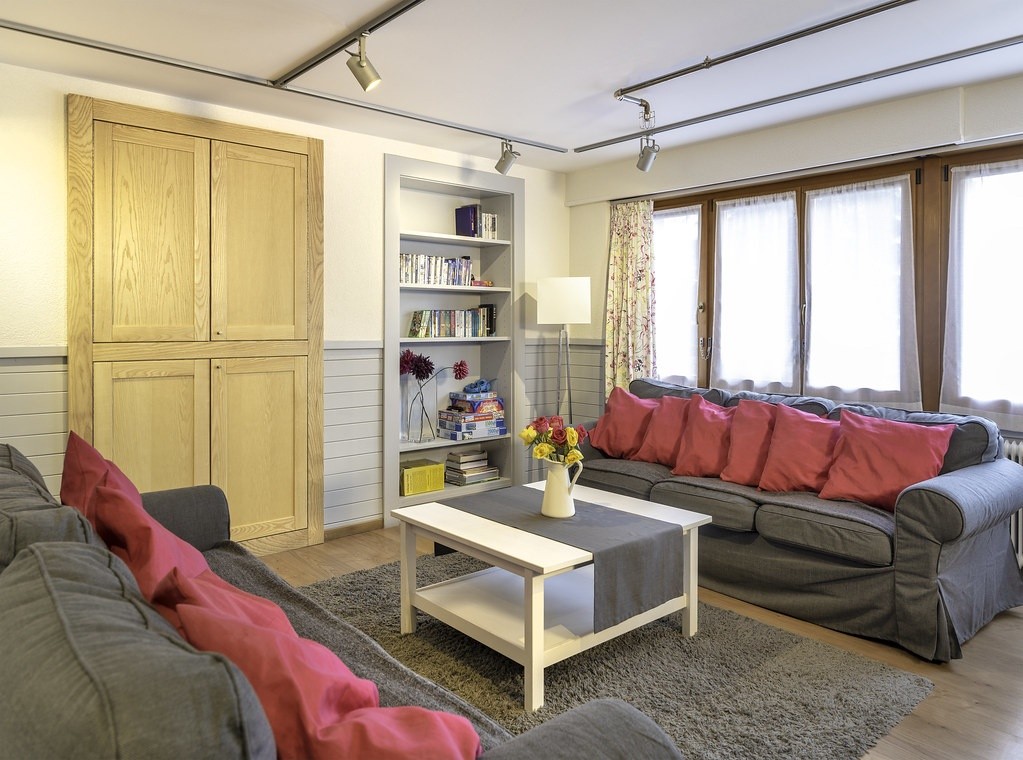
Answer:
[436,392,507,440]
[399,459,444,496]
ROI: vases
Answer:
[407,370,438,444]
[542,457,583,518]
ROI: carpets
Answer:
[295,552,934,759]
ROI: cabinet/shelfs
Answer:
[384,153,524,524]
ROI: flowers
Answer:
[519,415,587,465]
[400,350,470,443]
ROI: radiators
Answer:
[1003,439,1023,571]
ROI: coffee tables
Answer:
[389,479,712,714]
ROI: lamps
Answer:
[345,32,382,93]
[537,278,591,425]
[635,136,660,171]
[495,140,520,175]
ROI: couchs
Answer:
[547,378,1023,665]
[0,441,682,759]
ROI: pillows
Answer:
[60,431,483,760]
[587,387,956,512]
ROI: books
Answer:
[435,392,508,441]
[444,449,500,486]
[408,303,497,337]
[455,204,497,240]
[400,253,487,286]
